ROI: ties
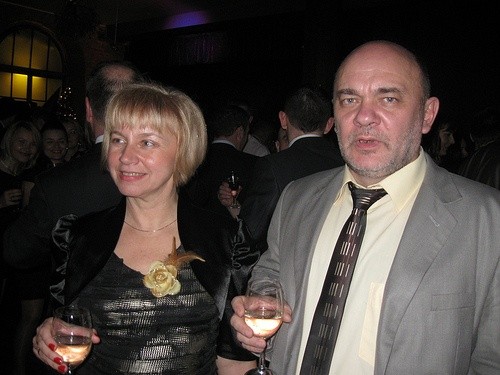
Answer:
[299,181,388,375]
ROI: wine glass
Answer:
[226,170,243,209]
[9,185,25,220]
[243,278,284,375]
[52,305,93,375]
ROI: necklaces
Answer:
[125,218,176,232]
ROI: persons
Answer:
[178,87,343,322]
[421,112,499,193]
[33,83,258,375]
[0,63,144,375]
[231,40,500,375]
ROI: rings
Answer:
[38,348,41,354]
